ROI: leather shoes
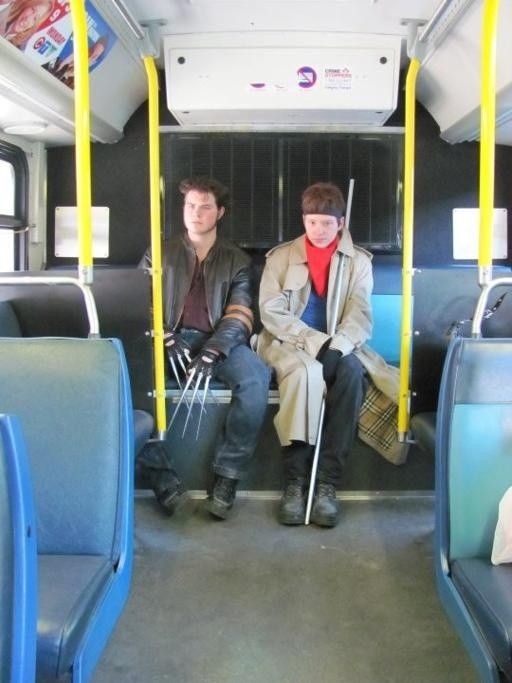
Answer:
[203,474,238,522]
[276,479,306,525]
[152,476,192,517]
[310,479,342,528]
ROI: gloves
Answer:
[163,334,192,359]
[187,350,219,384]
[321,348,341,385]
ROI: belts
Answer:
[178,328,204,334]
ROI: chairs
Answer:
[362,265,512,396]
[2,296,153,463]
[433,273,512,683]
[1,334,137,683]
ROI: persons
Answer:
[1,1,57,48]
[43,32,110,92]
[136,177,275,521]
[259,182,374,529]
